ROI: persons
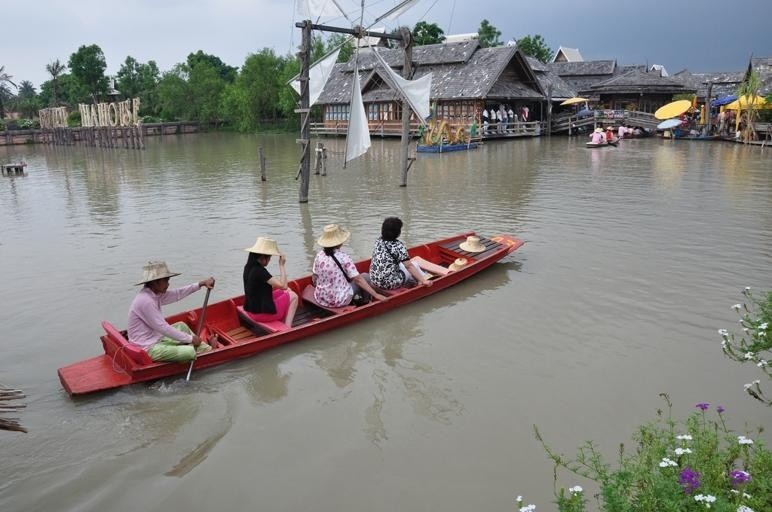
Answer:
[586,117,641,147]
[126,260,220,364]
[243,237,299,329]
[481,104,531,136]
[313,221,388,308]
[368,216,432,288]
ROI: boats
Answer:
[58,230,525,399]
[585,137,621,148]
[657,131,721,140]
[718,135,772,146]
[616,130,655,138]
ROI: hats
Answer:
[448,258,469,272]
[134,260,183,286]
[243,236,285,256]
[424,273,440,280]
[595,126,613,132]
[317,224,352,248]
[459,236,487,253]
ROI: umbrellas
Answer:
[658,121,682,140]
[724,93,772,133]
[559,95,589,120]
[713,92,742,136]
[654,100,692,120]
